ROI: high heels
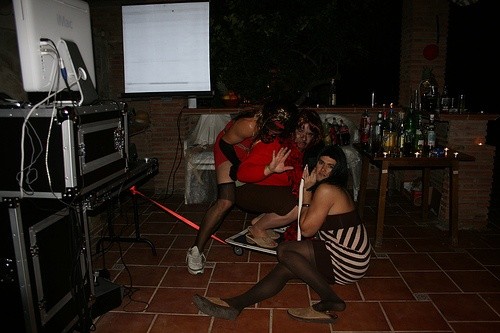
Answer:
[246,233,279,249]
[248,226,281,240]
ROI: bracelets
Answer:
[302,204,309,207]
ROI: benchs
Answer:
[184,113,360,205]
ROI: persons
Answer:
[194,144,372,322]
[186,99,324,275]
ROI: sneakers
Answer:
[185,246,206,275]
[272,225,290,233]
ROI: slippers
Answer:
[192,294,238,320]
[286,306,338,322]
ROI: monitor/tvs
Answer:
[12,0,98,108]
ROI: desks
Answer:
[87,168,158,255]
[353,143,476,249]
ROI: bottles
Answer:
[323,117,351,147]
[359,65,465,158]
[328,75,338,108]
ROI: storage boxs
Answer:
[0,103,158,333]
[401,179,433,208]
